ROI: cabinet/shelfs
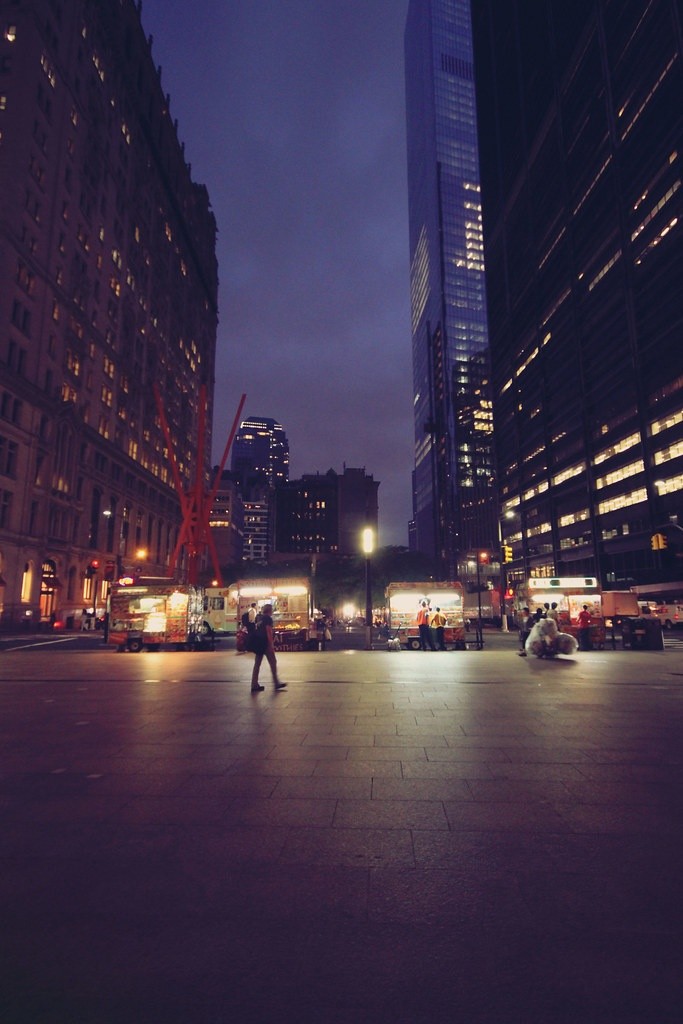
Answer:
[271,610,309,629]
[390,611,464,629]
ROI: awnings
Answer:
[43,577,64,589]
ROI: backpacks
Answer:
[245,616,265,652]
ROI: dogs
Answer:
[385,638,402,651]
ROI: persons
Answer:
[518,607,534,656]
[79,609,89,632]
[250,603,286,691]
[417,600,448,650]
[575,604,592,650]
[247,602,258,651]
[533,602,561,633]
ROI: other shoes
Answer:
[275,683,286,689]
[519,651,527,656]
[251,686,264,691]
